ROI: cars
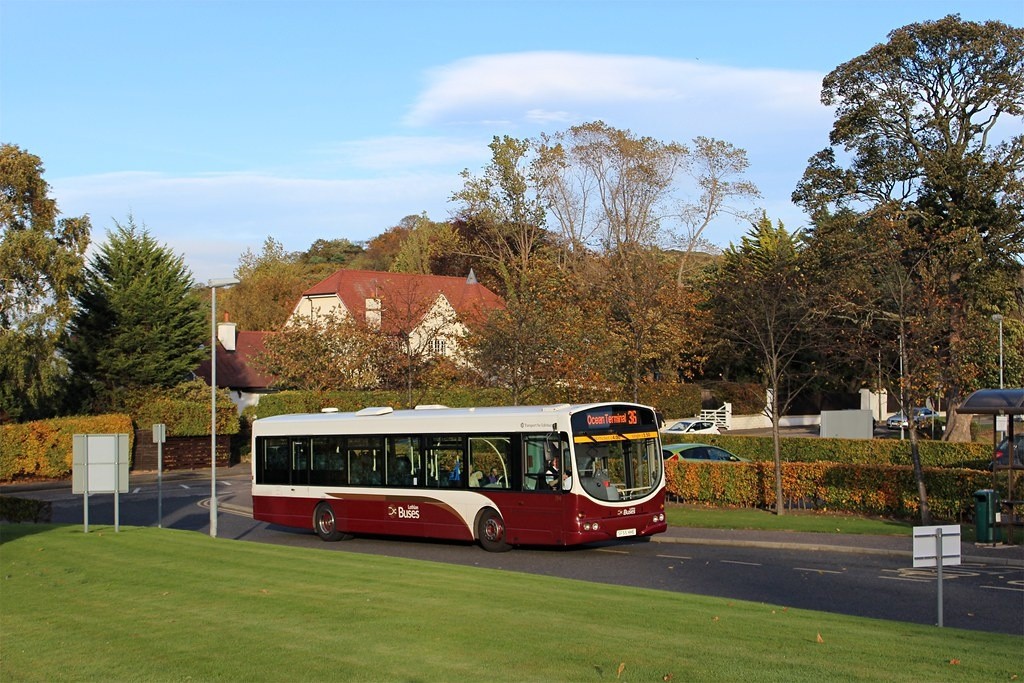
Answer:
[643,444,753,464]
[661,420,721,436]
[886,407,939,430]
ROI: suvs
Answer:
[988,433,1024,473]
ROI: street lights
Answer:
[207,276,241,537]
[991,314,1003,443]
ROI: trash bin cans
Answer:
[973,489,1003,545]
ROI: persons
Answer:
[544,457,571,491]
[488,467,499,483]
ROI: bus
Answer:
[251,404,668,553]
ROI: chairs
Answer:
[471,471,484,486]
[537,466,550,489]
